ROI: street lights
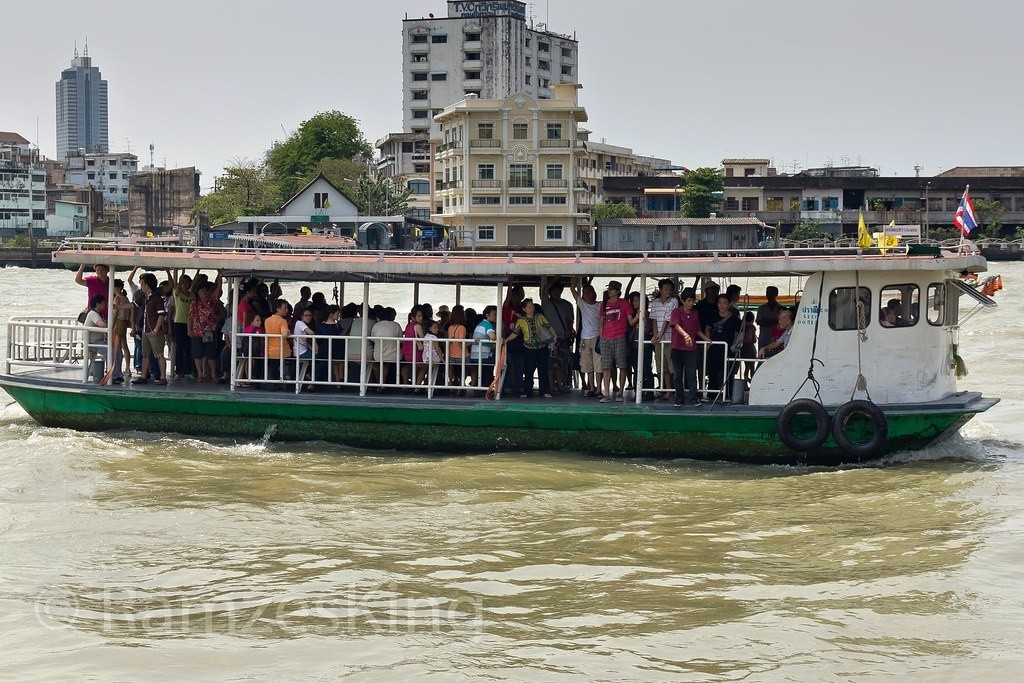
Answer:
[674,185,680,218]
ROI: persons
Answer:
[828,289,919,330]
[239,244,292,254]
[75,261,801,407]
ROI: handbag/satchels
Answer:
[540,324,554,345]
[415,334,423,351]
[77,310,87,324]
[241,327,260,357]
[203,332,213,342]
[595,335,603,355]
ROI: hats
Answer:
[606,281,621,290]
[437,304,451,317]
[704,280,716,290]
[93,263,110,272]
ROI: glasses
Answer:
[607,288,617,290]
[304,314,312,316]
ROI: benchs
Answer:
[234,355,495,395]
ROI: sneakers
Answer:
[191,372,197,379]
[177,374,185,380]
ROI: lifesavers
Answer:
[778,399,831,449]
[831,399,889,454]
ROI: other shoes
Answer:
[544,394,552,400]
[156,378,167,386]
[304,375,313,392]
[131,377,148,384]
[625,386,634,390]
[112,377,124,384]
[688,400,702,407]
[674,399,686,407]
[197,377,203,381]
[599,392,624,402]
[520,395,528,400]
[457,389,467,397]
[216,377,226,384]
[336,379,344,393]
[584,390,603,398]
[654,397,676,403]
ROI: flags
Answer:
[953,185,978,237]
[857,207,875,250]
[878,220,898,255]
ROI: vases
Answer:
[1019,243,1024,249]
[1001,243,1008,249]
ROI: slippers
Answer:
[237,384,252,388]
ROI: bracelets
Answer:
[636,314,639,319]
[767,346,771,351]
[154,329,158,333]
[684,335,690,339]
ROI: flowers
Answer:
[1002,238,1007,242]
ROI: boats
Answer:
[0,239,1001,465]
[226,232,357,283]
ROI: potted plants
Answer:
[822,239,830,247]
[849,239,856,247]
[835,239,841,247]
[794,240,802,247]
[982,240,989,248]
[807,241,814,248]
